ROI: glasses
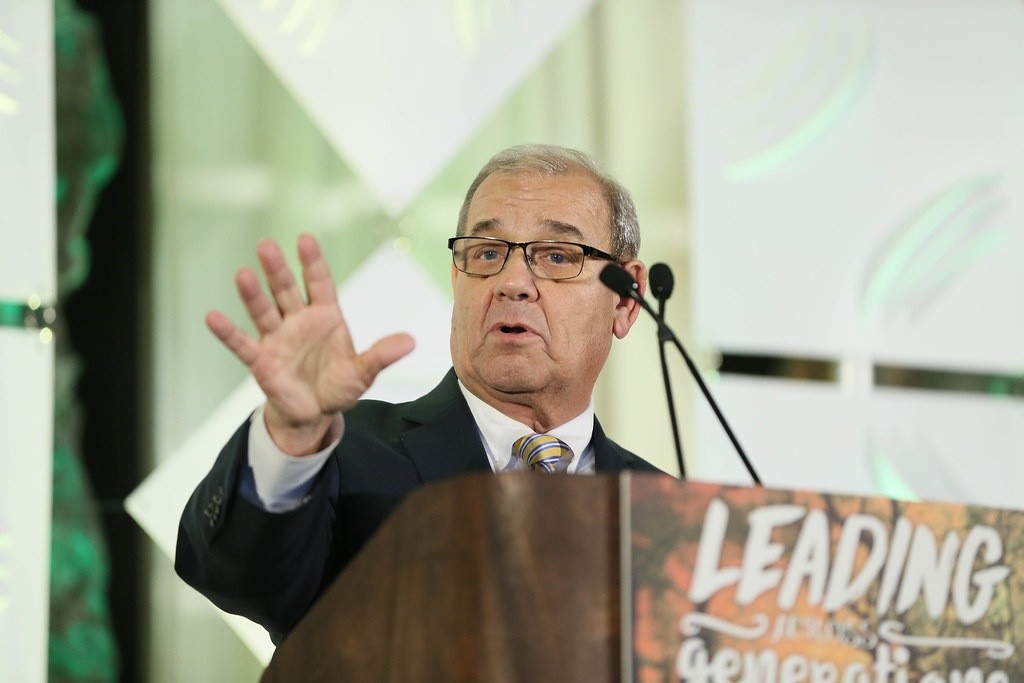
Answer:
[449,236,627,280]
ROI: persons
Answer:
[173,143,675,650]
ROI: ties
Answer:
[512,434,571,473]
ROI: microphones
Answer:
[598,262,765,487]
